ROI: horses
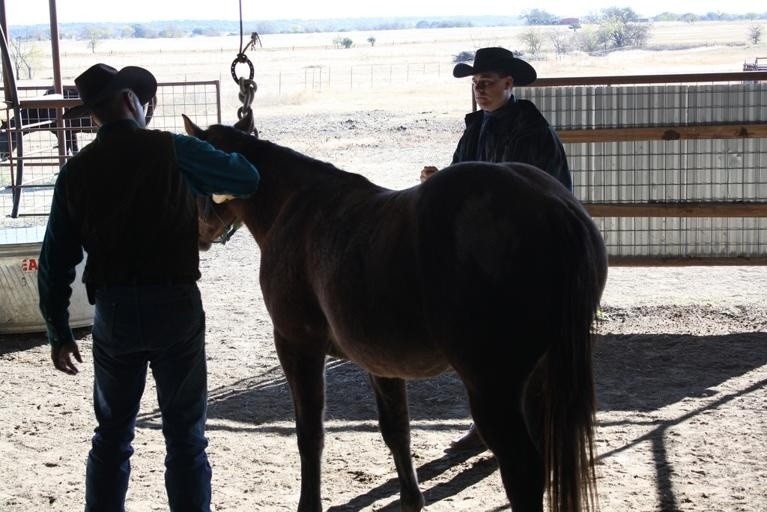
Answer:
[180,113,609,512]
[0,88,157,161]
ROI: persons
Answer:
[420,47,572,456]
[37,63,260,512]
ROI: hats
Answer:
[453,47,537,87]
[61,63,157,119]
